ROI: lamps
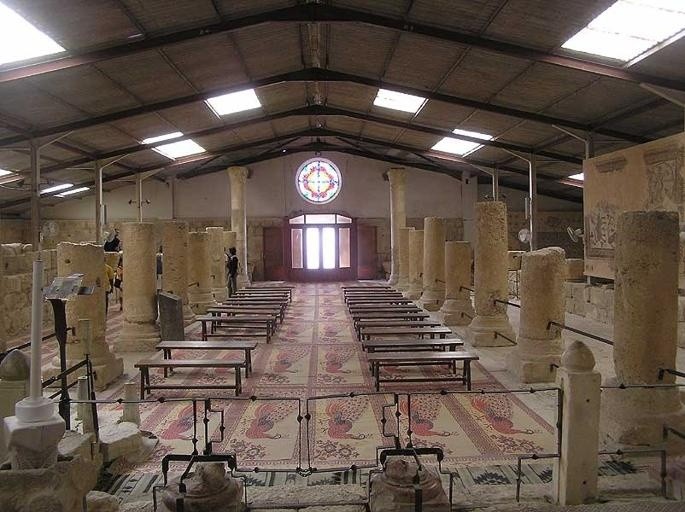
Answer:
[17,179,32,188]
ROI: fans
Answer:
[566,227,585,244]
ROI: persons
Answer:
[156,245,162,294]
[227,246,239,294]
[104,257,115,315]
[117,256,122,311]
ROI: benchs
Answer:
[340,286,480,392]
[134,285,296,401]
